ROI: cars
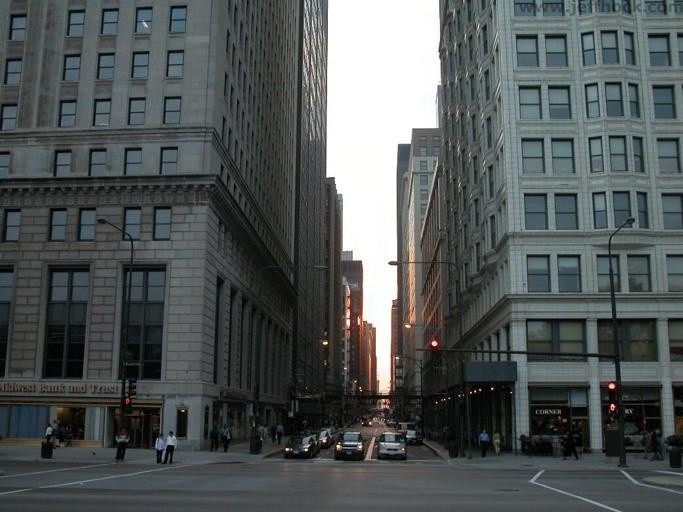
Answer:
[360,415,398,427]
[404,429,423,445]
[282,425,338,458]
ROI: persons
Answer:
[164,431,177,464]
[478,428,584,460]
[209,422,283,452]
[155,433,164,464]
[115,426,130,460]
[45,416,74,449]
[640,430,665,461]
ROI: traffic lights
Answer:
[607,381,617,416]
[430,336,439,352]
[122,396,131,413]
[129,377,137,396]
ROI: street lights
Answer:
[95,217,134,429]
[404,324,450,424]
[292,338,362,421]
[388,260,472,459]
[606,217,636,468]
[393,356,424,436]
[249,263,328,454]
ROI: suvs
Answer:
[333,431,366,461]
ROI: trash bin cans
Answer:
[41,442,56,458]
[669,448,683,468]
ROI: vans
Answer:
[376,432,407,460]
[395,422,415,439]
